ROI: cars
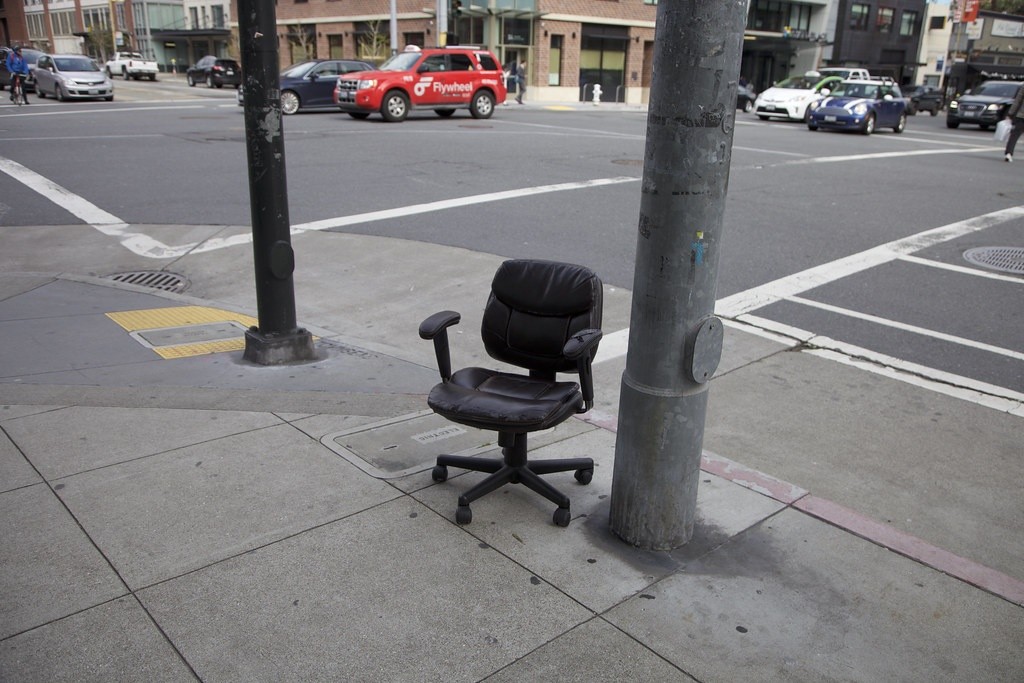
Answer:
[806,76,907,135]
[752,70,844,123]
[236,59,377,114]
[35,53,114,101]
[736,85,757,114]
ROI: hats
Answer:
[13,45,21,53]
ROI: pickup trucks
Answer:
[104,51,161,81]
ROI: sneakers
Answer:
[1005,153,1012,162]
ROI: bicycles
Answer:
[11,73,27,107]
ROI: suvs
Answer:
[333,44,508,122]
[947,79,1024,129]
[902,84,942,116]
[0,46,51,92]
[185,56,241,89]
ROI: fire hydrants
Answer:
[590,83,604,107]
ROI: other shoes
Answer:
[25,101,29,104]
[10,96,13,100]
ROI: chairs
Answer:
[418,260,604,529]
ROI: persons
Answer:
[6,44,31,104]
[513,60,527,104]
[1005,84,1024,161]
[501,64,510,106]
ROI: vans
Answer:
[815,68,871,83]
